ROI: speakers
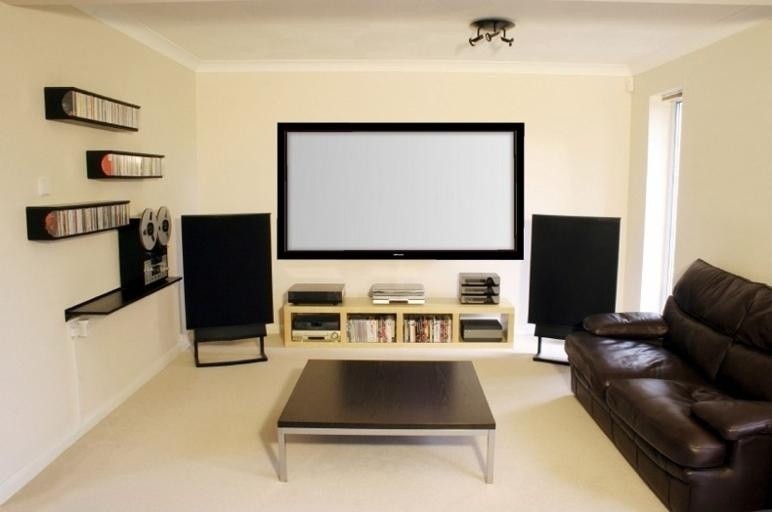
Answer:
[180,212,276,330]
[526,213,622,324]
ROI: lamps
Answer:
[468,16,515,49]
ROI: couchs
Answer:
[563,257,772,512]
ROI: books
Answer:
[46,204,132,237]
[103,155,163,177]
[66,92,139,128]
[347,318,451,344]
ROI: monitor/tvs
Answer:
[276,121,526,262]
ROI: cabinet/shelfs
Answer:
[283,297,515,351]
[24,85,183,322]
